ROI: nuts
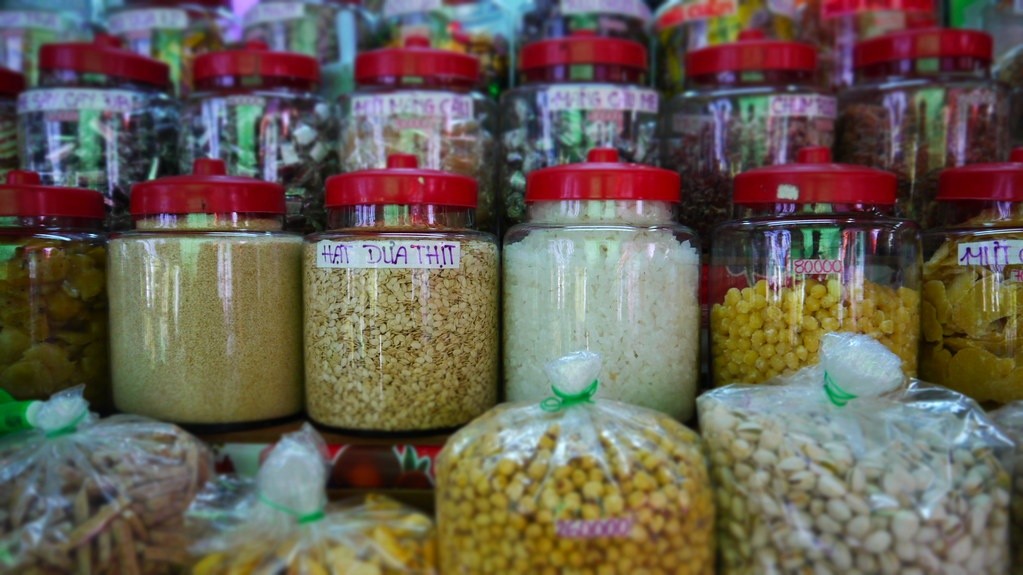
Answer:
[698,396,1012,574]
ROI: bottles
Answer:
[1,0,1023,444]
